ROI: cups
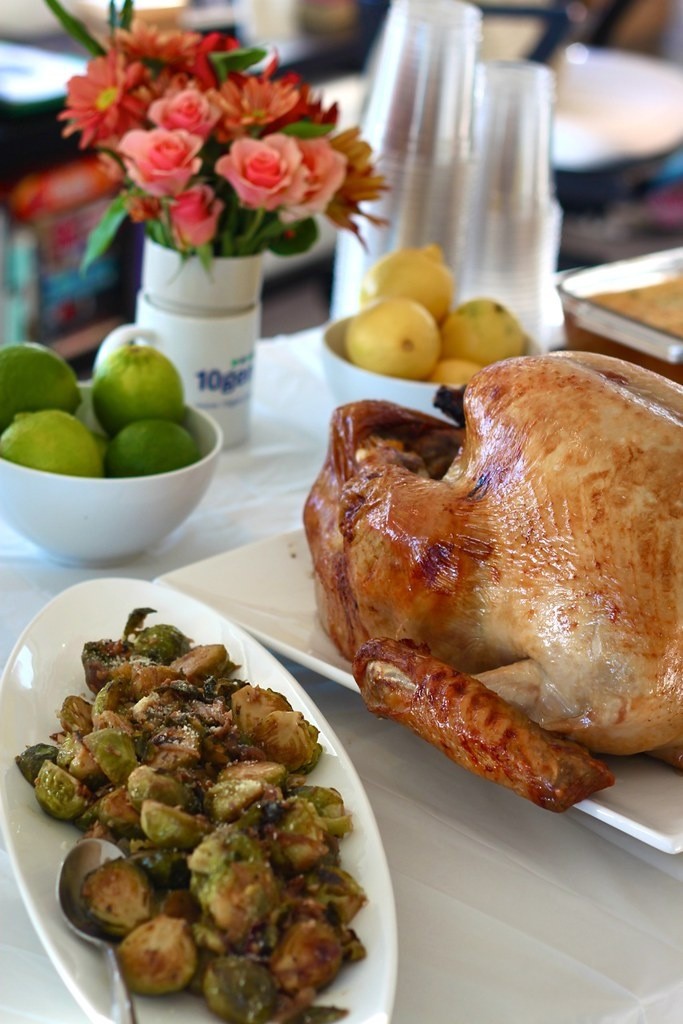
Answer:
[327,1,573,368]
[92,295,262,456]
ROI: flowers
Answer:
[42,0,393,283]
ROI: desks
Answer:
[0,279,683,1024]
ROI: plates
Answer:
[1,383,224,564]
[300,36,683,179]
[1,577,395,1024]
[158,530,682,855]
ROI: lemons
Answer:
[0,339,203,480]
[343,242,524,390]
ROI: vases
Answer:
[133,239,263,446]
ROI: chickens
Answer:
[303,351,683,812]
